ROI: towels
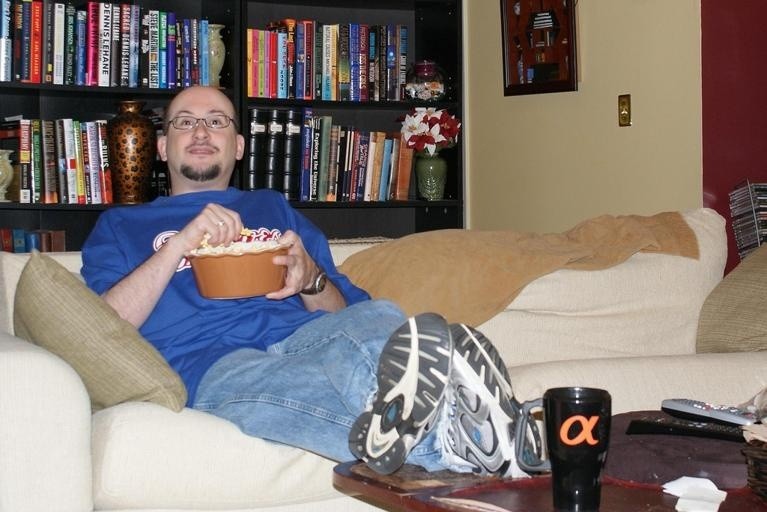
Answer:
[333,211,700,329]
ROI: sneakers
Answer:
[435,320,544,479]
[348,312,452,480]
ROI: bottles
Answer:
[404,57,447,106]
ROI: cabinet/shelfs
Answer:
[0,0,467,255]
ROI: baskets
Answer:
[740,439,767,500]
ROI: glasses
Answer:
[164,114,240,136]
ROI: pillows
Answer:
[14,248,189,413]
[695,244,766,353]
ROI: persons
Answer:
[80,84,556,481]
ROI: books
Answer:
[142,107,172,200]
[246,20,408,102]
[249,108,413,200]
[2,228,66,254]
[0,0,209,88]
[2,115,114,204]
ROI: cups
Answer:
[514,386,613,512]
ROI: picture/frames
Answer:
[499,0,578,97]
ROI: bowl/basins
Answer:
[182,242,297,302]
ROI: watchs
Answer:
[300,266,327,295]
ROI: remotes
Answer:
[626,415,745,442]
[662,399,763,427]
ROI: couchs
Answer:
[0,203,766,512]
